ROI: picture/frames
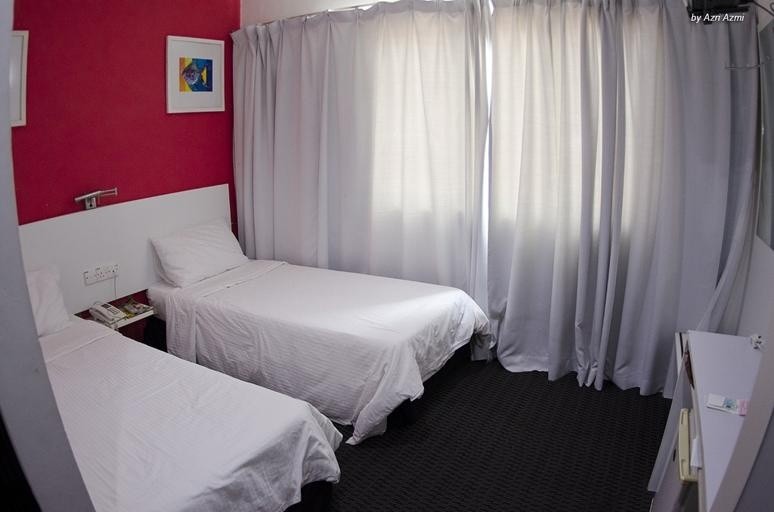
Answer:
[165,34,227,115]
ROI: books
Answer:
[705,394,749,416]
[114,297,155,331]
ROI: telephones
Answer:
[89,300,127,324]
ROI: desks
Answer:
[648,330,761,510]
